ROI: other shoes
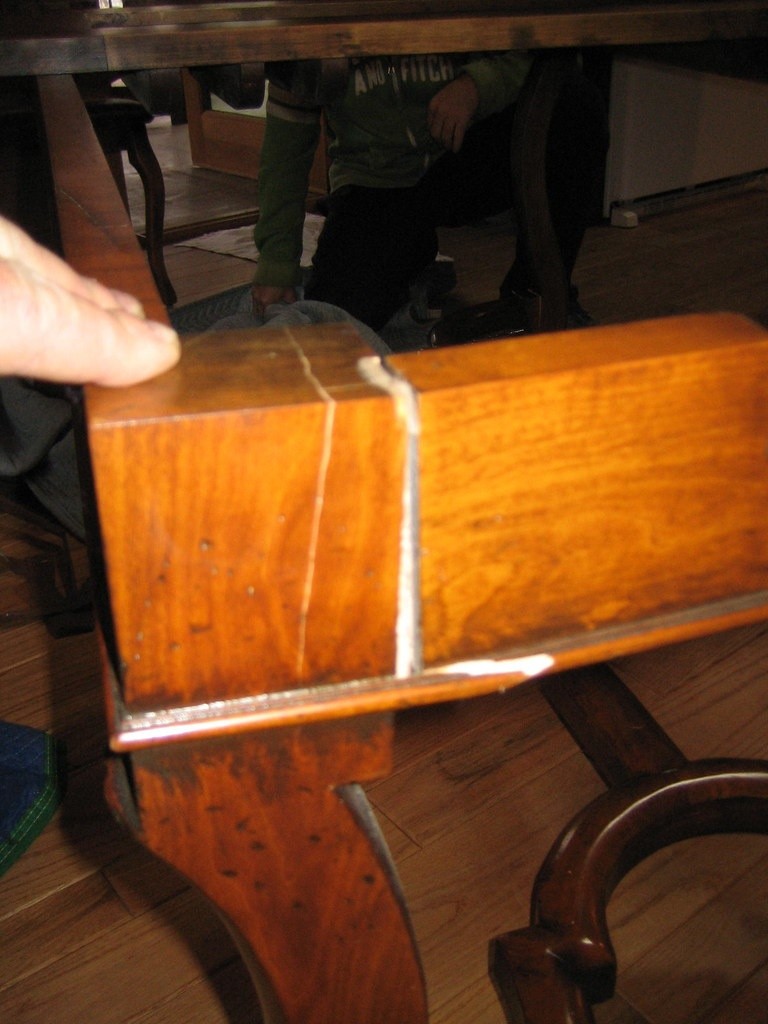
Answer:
[410,255,458,323]
[499,261,595,330]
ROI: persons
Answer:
[246,49,613,347]
[0,212,183,390]
